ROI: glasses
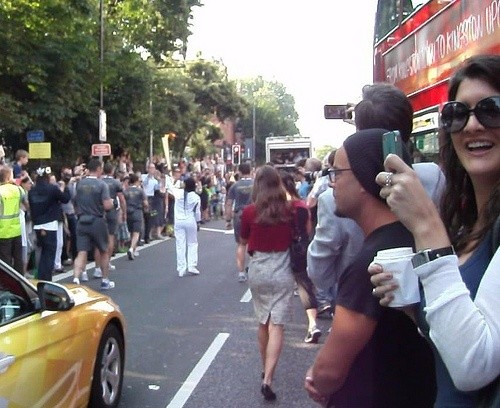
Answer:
[441,95,500,134]
[327,168,353,183]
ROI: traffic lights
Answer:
[232,145,241,166]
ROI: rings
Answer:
[371,289,376,295]
[385,172,393,187]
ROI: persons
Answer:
[70,158,115,290]
[367,56,500,408]
[304,128,440,408]
[229,150,338,342]
[237,167,312,399]
[165,174,202,277]
[304,82,447,313]
[101,158,125,271]
[109,154,165,261]
[155,157,229,237]
[1,150,102,285]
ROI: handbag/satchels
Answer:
[79,214,96,224]
[290,212,308,273]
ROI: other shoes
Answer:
[53,258,73,274]
[188,266,201,274]
[178,271,185,276]
[132,251,139,256]
[238,271,247,282]
[127,248,134,260]
[24,272,34,279]
[317,303,332,315]
[111,233,166,256]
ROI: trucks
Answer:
[266,136,312,174]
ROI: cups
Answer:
[374,246,422,307]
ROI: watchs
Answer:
[410,245,455,270]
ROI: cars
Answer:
[0,259,126,408]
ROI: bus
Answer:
[374,0,500,163]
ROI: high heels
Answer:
[261,384,275,400]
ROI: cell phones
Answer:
[324,104,352,119]
[383,129,401,173]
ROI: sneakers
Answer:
[80,275,89,281]
[92,270,103,278]
[101,281,114,290]
[108,263,116,271]
[304,327,322,343]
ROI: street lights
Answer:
[252,93,283,164]
[149,65,187,160]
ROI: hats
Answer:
[342,128,411,200]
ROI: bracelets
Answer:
[225,219,231,222]
